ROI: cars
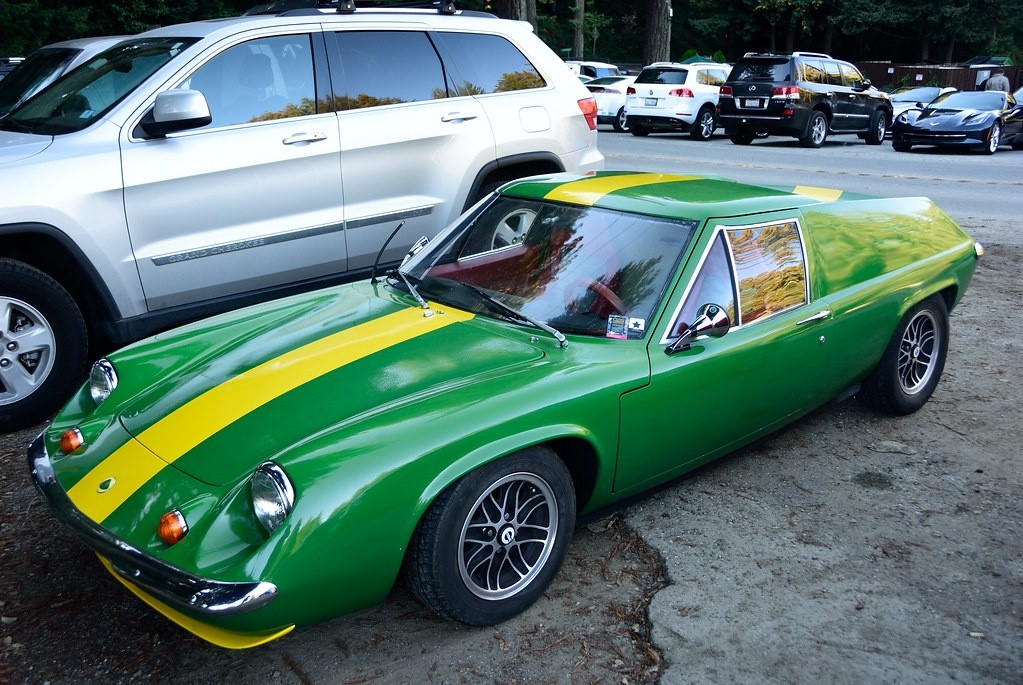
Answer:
[565,61,642,83]
[1012,86,1023,106]
[0,34,288,121]
[583,75,639,133]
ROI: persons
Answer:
[977,68,1011,93]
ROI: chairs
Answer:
[230,51,288,116]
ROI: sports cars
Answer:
[890,90,1023,156]
[856,84,957,139]
[24,169,988,651]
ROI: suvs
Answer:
[716,51,895,150]
[0,0,606,435]
[623,62,770,141]
